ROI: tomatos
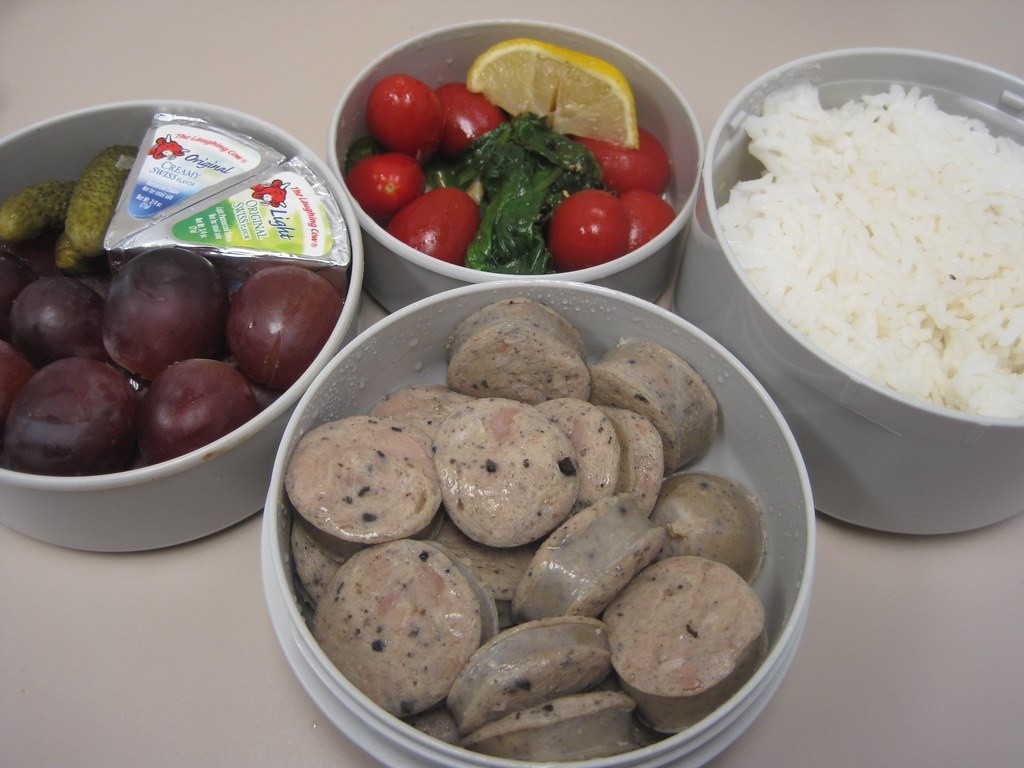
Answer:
[548,127,680,271]
[348,71,503,264]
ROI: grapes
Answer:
[0,247,340,475]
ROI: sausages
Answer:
[285,298,772,763]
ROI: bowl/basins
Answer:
[666,43,1023,536]
[0,98,364,554]
[328,20,704,316]
[259,278,817,768]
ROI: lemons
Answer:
[465,37,640,151]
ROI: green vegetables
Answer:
[341,110,616,275]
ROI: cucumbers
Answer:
[0,145,142,271]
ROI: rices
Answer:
[714,81,1024,422]
